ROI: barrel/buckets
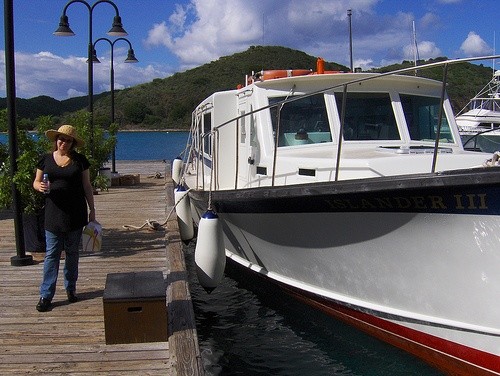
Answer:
[99,167,111,188]
[111,172,119,186]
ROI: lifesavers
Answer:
[254,68,311,82]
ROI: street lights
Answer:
[347,7,355,73]
[52,0,128,195]
[84,37,139,176]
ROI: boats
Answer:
[171,67,500,376]
[450,66,500,127]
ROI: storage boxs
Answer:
[103,270,168,345]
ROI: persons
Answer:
[33,125,96,311]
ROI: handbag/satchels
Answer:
[83,220,103,252]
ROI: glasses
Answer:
[58,137,74,143]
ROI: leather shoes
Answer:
[67,290,77,302]
[36,297,51,311]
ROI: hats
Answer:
[45,124,85,147]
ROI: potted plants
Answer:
[0,110,119,252]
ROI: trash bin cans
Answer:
[99,168,111,188]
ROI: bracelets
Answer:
[90,206,95,211]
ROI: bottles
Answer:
[42,174,50,194]
